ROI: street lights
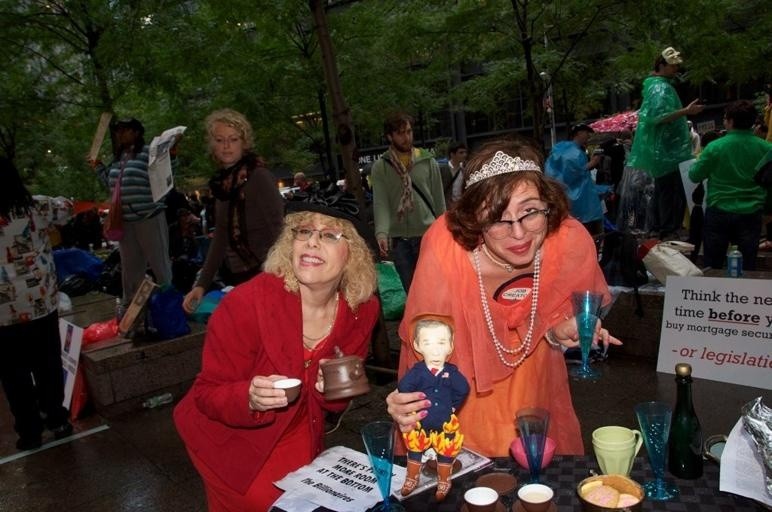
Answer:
[642,241,704,286]
[145,286,192,342]
[102,184,126,241]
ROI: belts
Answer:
[515,407,550,485]
[360,420,406,512]
[633,400,681,502]
[567,291,604,380]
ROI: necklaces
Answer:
[474,244,542,368]
[303,293,339,351]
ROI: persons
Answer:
[686,100,771,272]
[370,114,446,293]
[35,201,115,252]
[294,172,309,191]
[628,47,705,245]
[171,181,381,512]
[544,124,617,265]
[84,117,181,304]
[582,134,632,224]
[2,163,73,450]
[437,141,467,208]
[183,109,283,313]
[166,181,216,263]
[385,136,623,458]
[397,312,470,501]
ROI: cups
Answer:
[486,208,554,240]
[290,225,341,242]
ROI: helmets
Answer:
[425,457,461,473]
[460,499,507,512]
[476,472,517,494]
[511,498,558,512]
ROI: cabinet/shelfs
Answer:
[726,245,743,278]
[668,362,704,480]
[142,392,174,409]
[114,298,124,322]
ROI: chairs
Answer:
[16,438,41,449]
[54,423,73,439]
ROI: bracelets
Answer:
[545,316,569,346]
[510,435,556,470]
[576,474,645,512]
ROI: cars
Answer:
[517,483,554,512]
[272,378,302,403]
[463,486,499,512]
[592,425,643,478]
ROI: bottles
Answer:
[284,177,370,238]
[571,124,594,136]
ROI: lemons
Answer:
[321,345,372,402]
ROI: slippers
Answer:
[594,231,649,286]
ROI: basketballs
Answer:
[584,473,642,508]
